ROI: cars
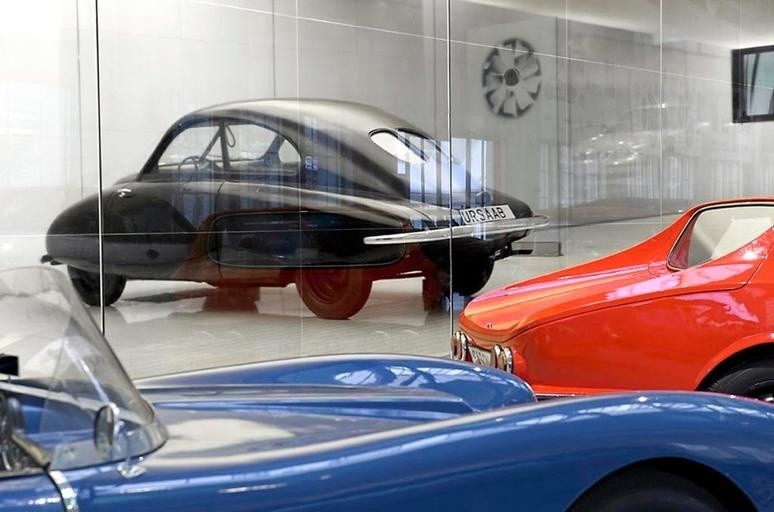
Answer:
[0,263,774,512]
[451,197,774,407]
[40,96,552,320]
